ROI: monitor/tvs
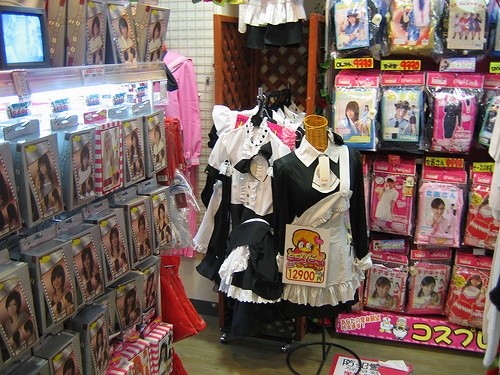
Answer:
[1,5,51,70]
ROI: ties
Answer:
[392,121,399,139]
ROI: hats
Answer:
[394,100,410,109]
[385,175,396,183]
[346,9,359,17]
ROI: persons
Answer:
[340,101,370,136]
[36,154,54,197]
[94,327,104,360]
[137,215,149,245]
[3,291,29,338]
[370,277,400,308]
[88,16,102,50]
[417,276,444,307]
[50,264,70,307]
[449,274,483,320]
[78,146,91,184]
[339,9,365,43]
[107,227,124,263]
[63,358,75,375]
[145,272,155,298]
[80,247,98,285]
[123,289,138,319]
[129,130,140,165]
[160,342,168,370]
[103,130,116,178]
[271,114,372,319]
[451,12,482,40]
[153,126,164,155]
[387,100,416,134]
[149,22,161,53]
[375,176,399,223]
[158,203,168,233]
[429,198,456,237]
[117,16,134,52]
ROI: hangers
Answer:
[254,88,306,142]
